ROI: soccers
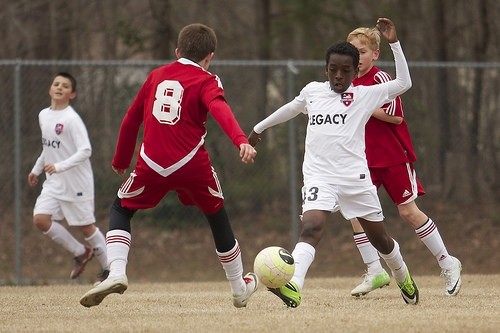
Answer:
[253,246,295,288]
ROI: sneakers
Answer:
[350,268,390,297]
[79,275,129,308]
[70,244,95,280]
[266,280,302,308]
[93,270,110,285]
[440,256,462,296]
[395,265,420,306]
[231,272,259,308]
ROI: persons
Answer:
[247,15,420,307]
[345,26,463,299]
[78,24,257,310]
[27,72,113,288]
